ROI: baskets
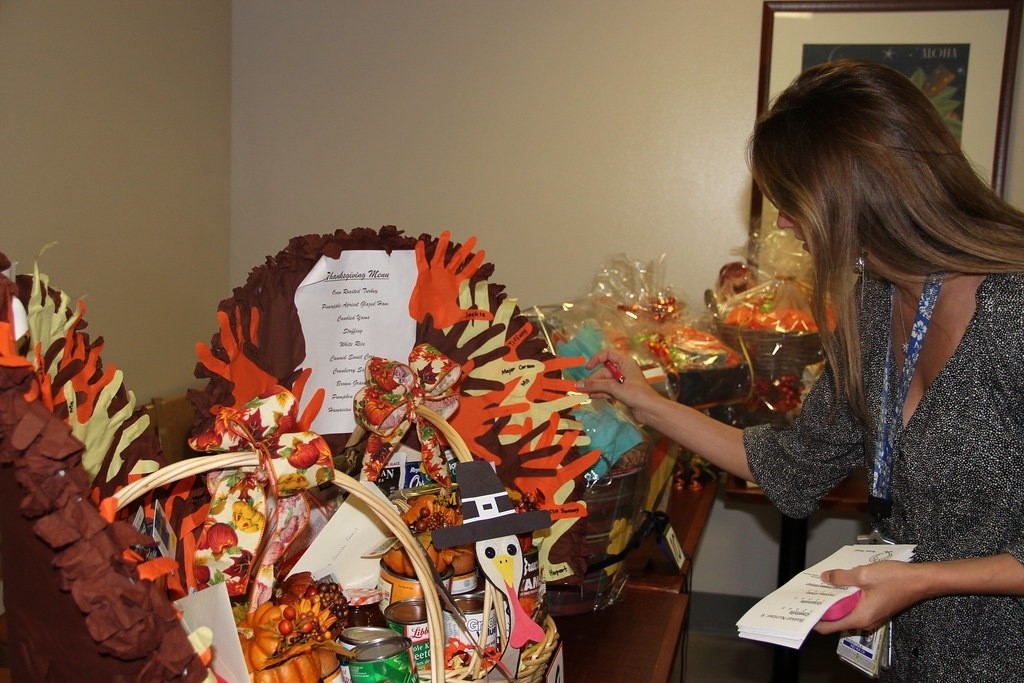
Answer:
[542,463,642,616]
[705,289,832,381]
[99,402,559,683]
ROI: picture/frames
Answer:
[748,0,1024,248]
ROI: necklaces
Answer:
[897,286,910,355]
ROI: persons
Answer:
[576,61,1024,683]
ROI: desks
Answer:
[725,466,870,683]
[617,467,723,594]
[550,591,691,683]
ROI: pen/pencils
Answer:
[604,359,626,384]
